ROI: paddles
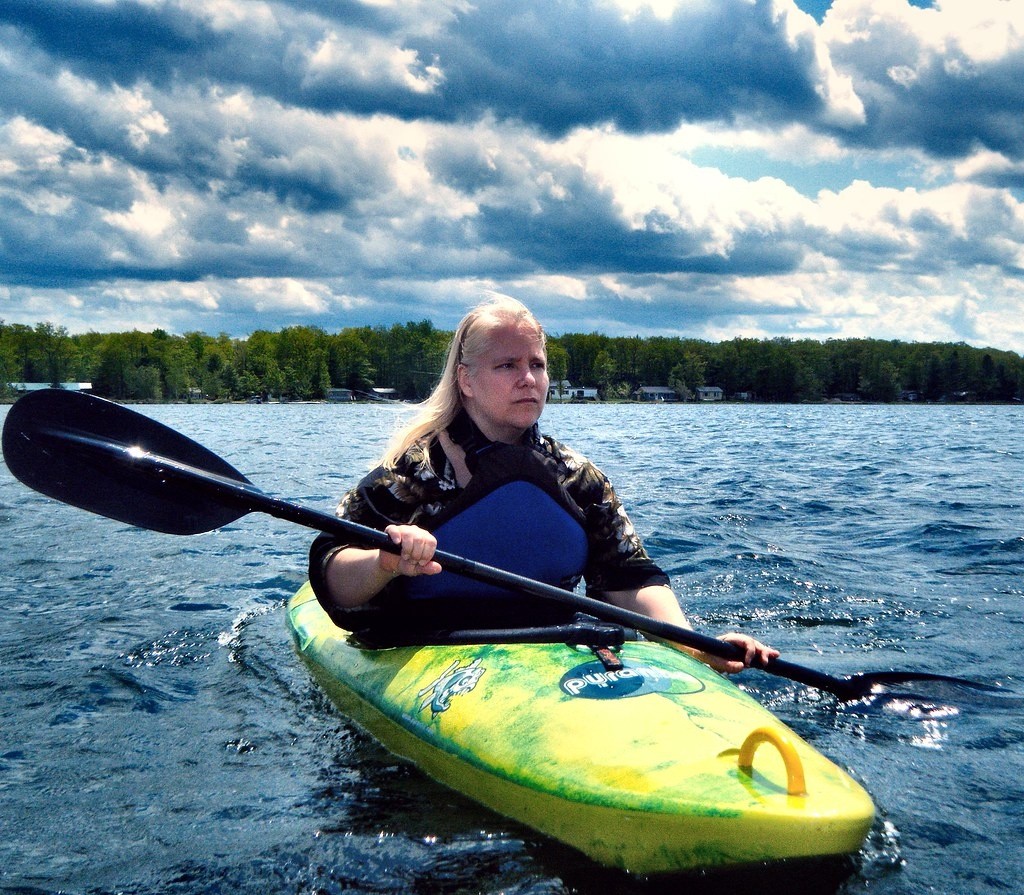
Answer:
[1,389,1015,703]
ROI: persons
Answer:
[308,295,779,674]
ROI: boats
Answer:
[284,576,875,895]
